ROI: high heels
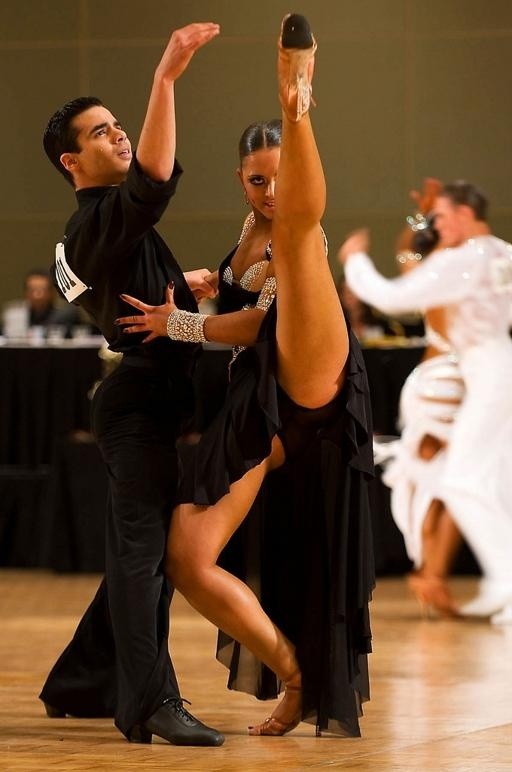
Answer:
[120,695,224,749]
[406,566,458,623]
[246,672,325,737]
[274,10,319,121]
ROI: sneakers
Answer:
[456,577,512,621]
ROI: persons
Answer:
[338,177,512,625]
[369,181,471,614]
[41,21,227,745]
[114,13,373,743]
[23,267,102,338]
[338,277,403,337]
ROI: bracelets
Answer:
[166,309,212,343]
